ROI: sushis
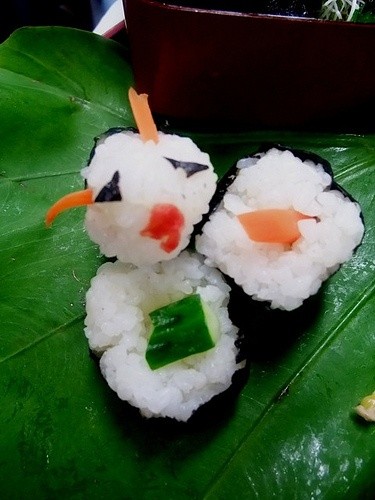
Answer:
[44,87,368,424]
